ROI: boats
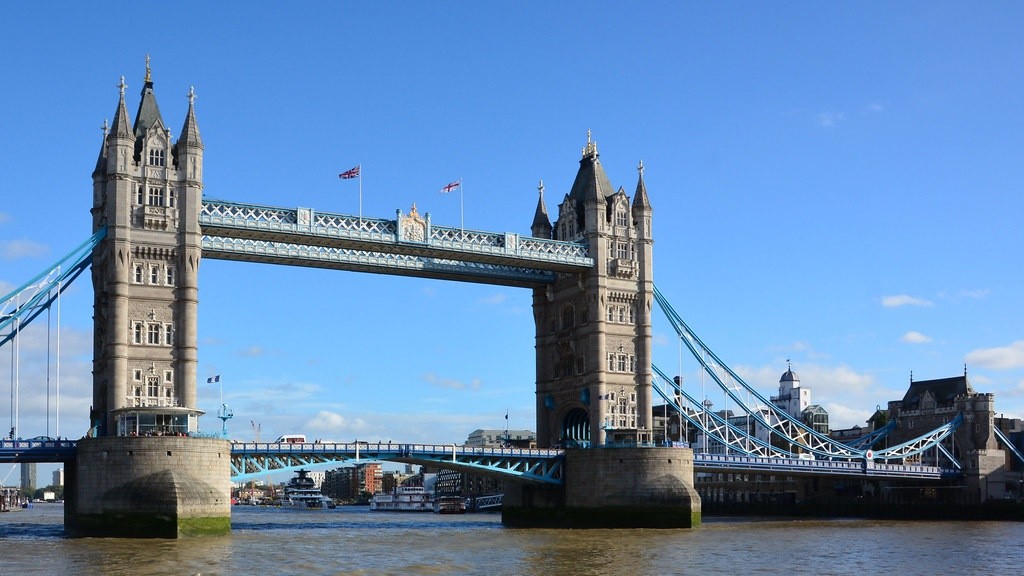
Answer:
[368,485,435,512]
[280,468,337,510]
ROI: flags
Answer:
[207,375,219,383]
[442,181,460,192]
[339,165,359,179]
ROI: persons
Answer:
[131,429,187,437]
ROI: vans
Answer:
[275,434,306,442]
